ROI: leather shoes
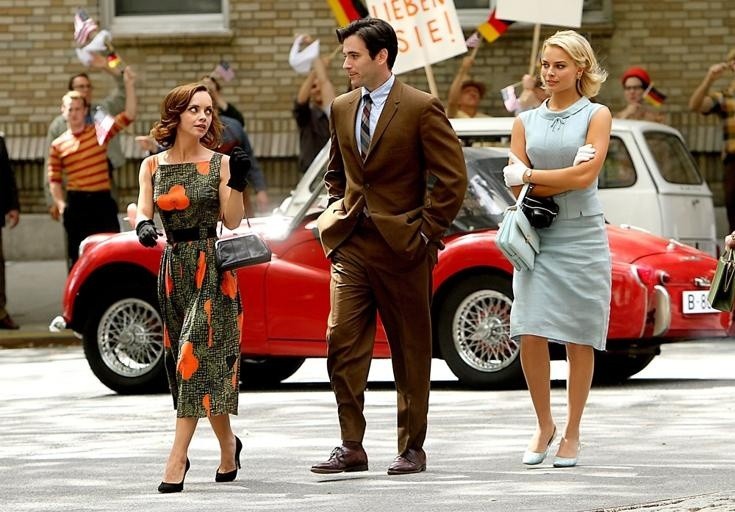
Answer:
[311,446,368,473]
[388,448,426,474]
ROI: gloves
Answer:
[136,219,162,247]
[503,152,527,187]
[573,144,595,166]
[226,147,250,191]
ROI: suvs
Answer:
[218,115,720,260]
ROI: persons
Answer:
[0,55,136,333]
[308,15,470,477]
[295,32,359,181]
[491,27,616,470]
[132,80,251,495]
[445,56,489,118]
[133,76,267,216]
[515,75,547,116]
[617,69,670,124]
[688,50,735,252]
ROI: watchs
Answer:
[524,167,533,184]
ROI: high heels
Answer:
[553,438,580,467]
[216,435,242,482]
[158,457,189,493]
[523,426,557,465]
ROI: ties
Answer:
[361,94,372,160]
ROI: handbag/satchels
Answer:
[707,248,735,312]
[215,213,271,272]
[496,206,540,271]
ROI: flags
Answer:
[212,61,235,81]
[330,1,367,27]
[70,8,120,68]
[465,7,514,48]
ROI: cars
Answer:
[45,146,727,396]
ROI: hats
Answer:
[461,74,486,98]
[622,67,650,85]
